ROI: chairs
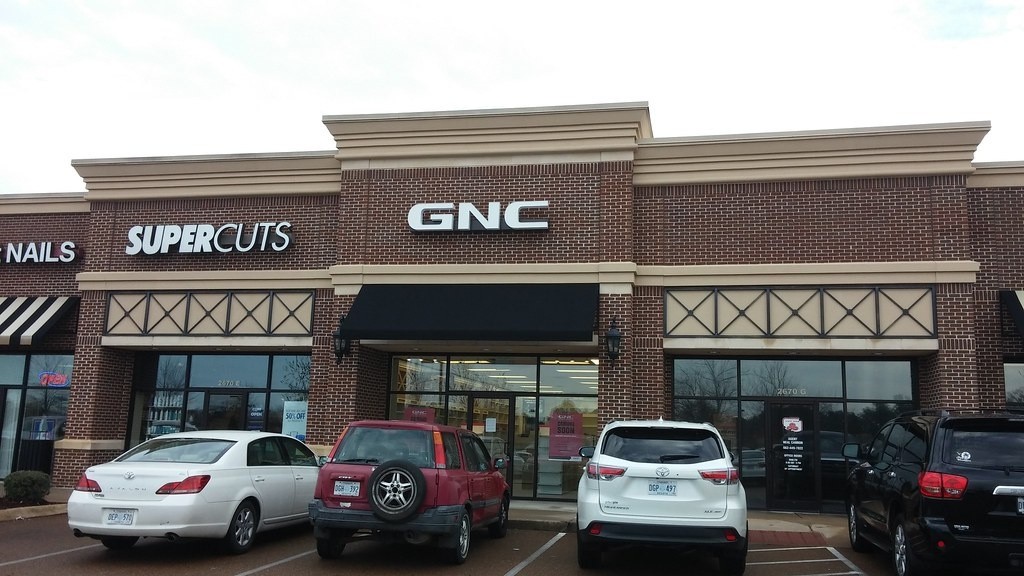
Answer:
[365,446,390,461]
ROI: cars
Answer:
[478,437,592,491]
[67,430,329,550]
[733,448,766,482]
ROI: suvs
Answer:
[574,415,748,576]
[771,432,860,504]
[841,408,1024,576]
[306,419,513,564]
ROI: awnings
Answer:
[0,296,80,345]
[339,282,599,342]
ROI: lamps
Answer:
[605,313,622,368]
[332,315,351,365]
